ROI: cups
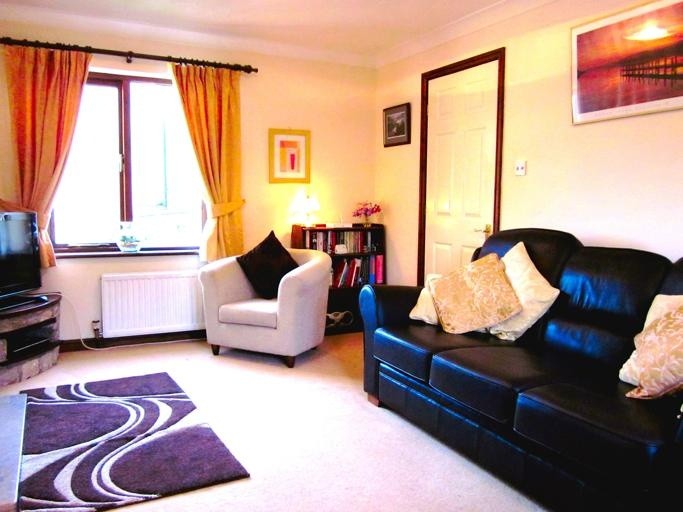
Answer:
[289,153,296,170]
[338,216,343,226]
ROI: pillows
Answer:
[619,294,683,386]
[236,230,298,299]
[626,304,683,399]
[409,273,443,326]
[487,241,560,341]
[427,253,523,334]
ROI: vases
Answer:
[363,219,371,228]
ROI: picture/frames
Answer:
[269,129,311,184]
[383,103,411,147]
[570,1,682,125]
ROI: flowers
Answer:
[351,201,381,219]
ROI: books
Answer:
[305,230,336,255]
[335,254,384,288]
[334,231,372,253]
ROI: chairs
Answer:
[198,248,332,368]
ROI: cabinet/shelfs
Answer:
[290,224,387,336]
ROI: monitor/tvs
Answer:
[0,210,43,310]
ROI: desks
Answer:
[0,292,62,388]
[0,394,27,512]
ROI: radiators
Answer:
[100,272,206,338]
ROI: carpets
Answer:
[18,372,251,512]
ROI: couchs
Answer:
[359,228,683,512]
